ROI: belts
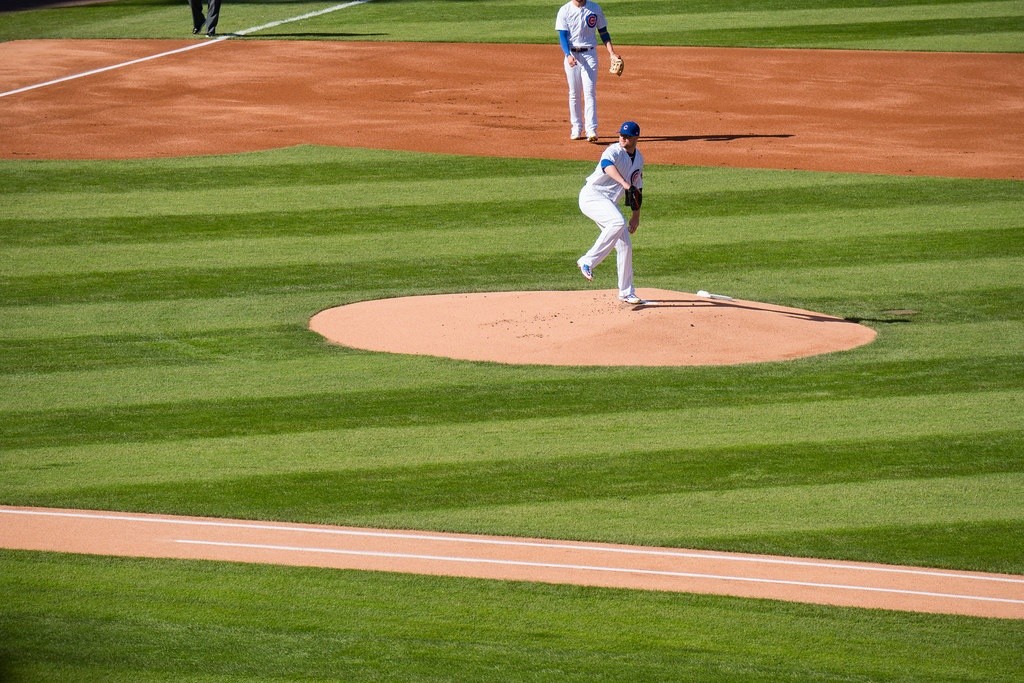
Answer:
[571,46,594,52]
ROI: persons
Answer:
[189,0,222,37]
[577,121,646,303]
[555,0,620,141]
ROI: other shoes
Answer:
[570,132,581,139]
[193,18,206,34]
[587,131,598,142]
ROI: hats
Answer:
[617,121,640,137]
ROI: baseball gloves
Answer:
[625,185,643,212]
[608,53,624,77]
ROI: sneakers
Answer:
[577,259,592,280]
[619,293,641,304]
